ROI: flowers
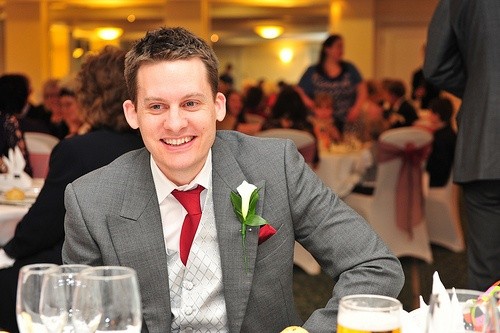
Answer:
[229,178,270,274]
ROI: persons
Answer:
[0,61,457,190]
[0,46,145,333]
[424,0,500,292]
[62,27,405,333]
[297,33,367,133]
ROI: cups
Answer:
[335,295,403,333]
[427,288,499,333]
[40,265,101,333]
[72,265,142,333]
[16,264,67,333]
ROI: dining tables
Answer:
[315,148,373,198]
[0,177,45,268]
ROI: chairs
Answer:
[0,126,464,277]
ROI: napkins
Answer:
[2,146,33,187]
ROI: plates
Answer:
[0,200,35,205]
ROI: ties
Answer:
[171,184,205,266]
